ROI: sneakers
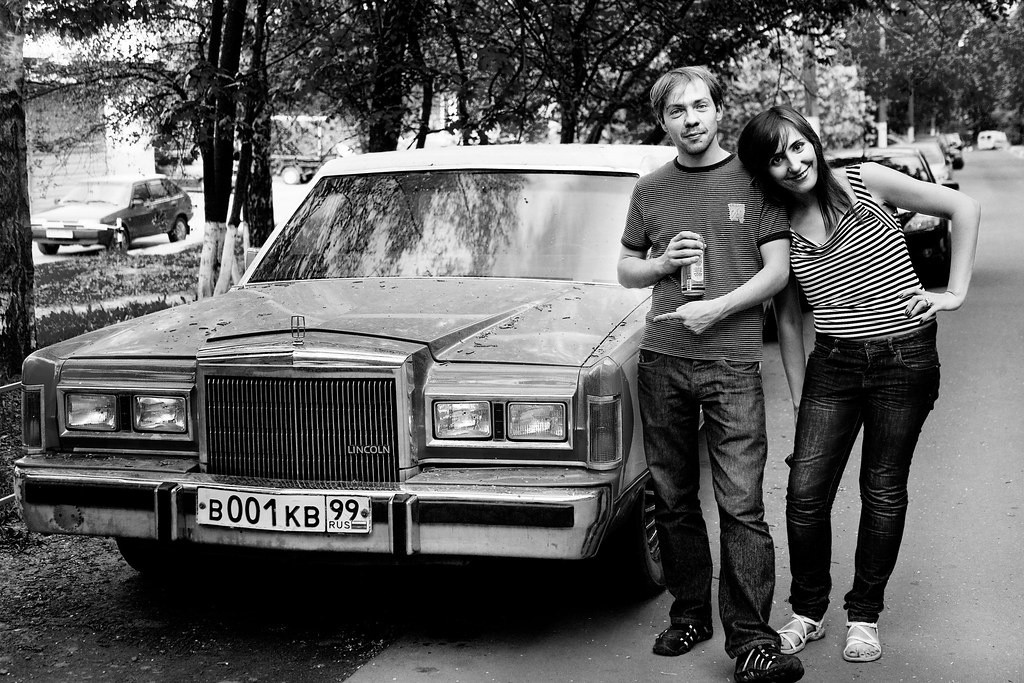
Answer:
[653,622,713,656]
[734,644,805,683]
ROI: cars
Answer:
[914,133,965,170]
[893,142,953,181]
[798,148,959,312]
[154,139,196,165]
[31,173,193,255]
[13,143,705,596]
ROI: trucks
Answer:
[179,117,339,185]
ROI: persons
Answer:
[617,67,804,683]
[737,106,982,662]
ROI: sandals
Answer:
[776,612,826,654]
[843,615,881,661]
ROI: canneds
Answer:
[681,234,706,296]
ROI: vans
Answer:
[978,131,1008,151]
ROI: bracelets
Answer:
[945,290,963,304]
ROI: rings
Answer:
[925,298,931,307]
